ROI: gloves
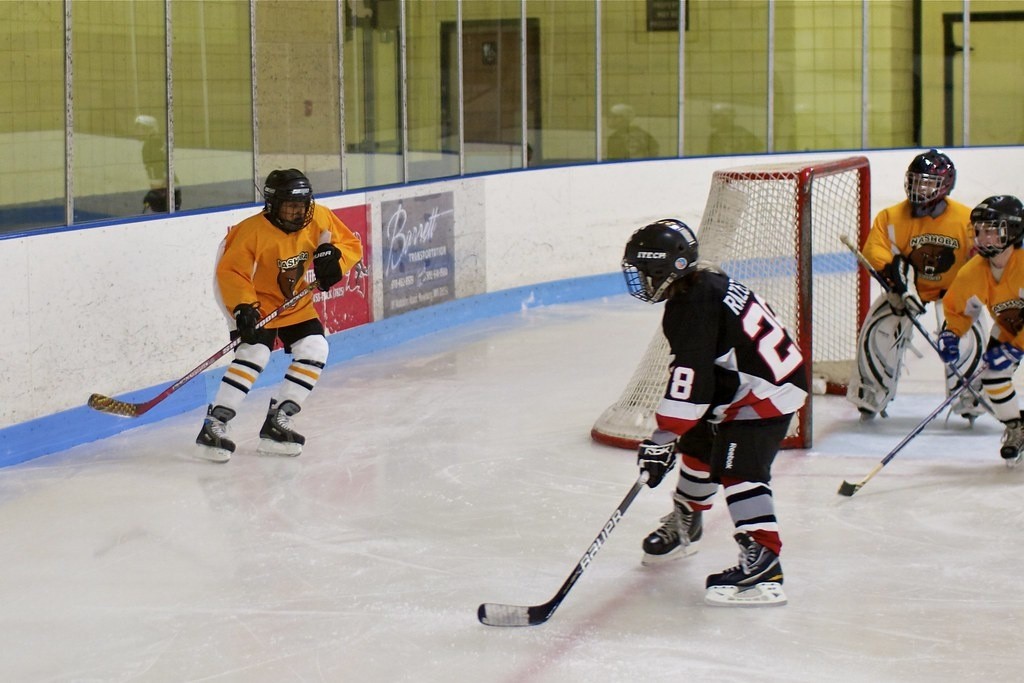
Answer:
[938,330,961,361]
[313,242,343,291]
[233,302,260,344]
[981,341,1024,371]
[637,438,677,488]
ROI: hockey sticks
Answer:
[87,279,318,418]
[476,470,651,627]
[837,363,990,496]
[840,236,996,417]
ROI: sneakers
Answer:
[857,407,875,422]
[963,413,978,428]
[194,404,236,462]
[704,532,787,605]
[256,397,305,456]
[1000,410,1024,467]
[641,490,703,565]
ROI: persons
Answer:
[937,195,1024,468]
[134,114,182,213]
[194,168,363,464]
[621,220,809,607]
[846,150,986,429]
[607,104,659,160]
[708,102,763,155]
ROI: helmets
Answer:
[904,152,955,205]
[967,195,1023,257]
[263,168,315,231]
[622,219,698,303]
